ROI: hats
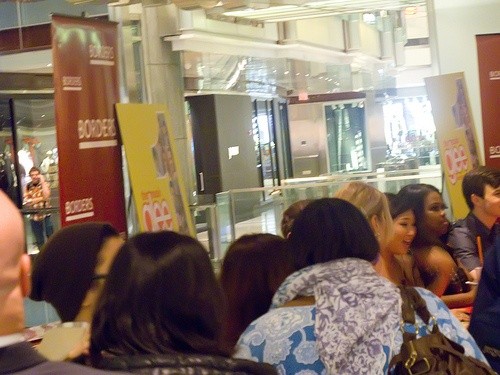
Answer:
[30,220,115,322]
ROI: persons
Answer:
[228,198,496,375]
[444,165,498,281]
[382,191,415,284]
[281,200,310,238]
[69,230,276,375]
[25,167,54,248]
[334,183,394,282]
[0,191,128,374]
[217,234,291,334]
[398,183,479,309]
[28,222,125,368]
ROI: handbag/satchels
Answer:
[388,286,498,375]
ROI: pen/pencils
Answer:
[465,281,480,286]
[475,235,484,266]
